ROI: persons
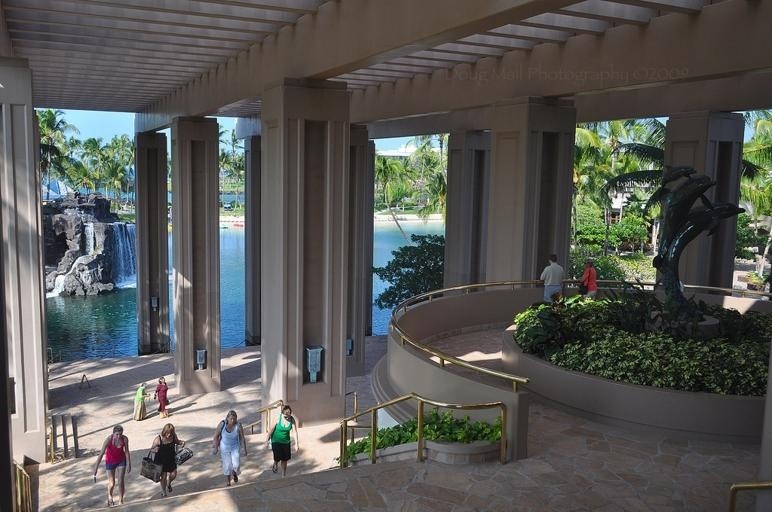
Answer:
[151,423,185,496]
[213,411,247,486]
[94,425,131,507]
[579,258,597,300]
[266,405,299,477]
[154,377,169,418]
[133,383,150,421]
[540,254,567,303]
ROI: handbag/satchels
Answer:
[140,448,163,483]
[174,444,193,465]
[578,284,588,295]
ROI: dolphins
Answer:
[663,203,746,295]
[659,163,697,198]
[652,174,717,265]
